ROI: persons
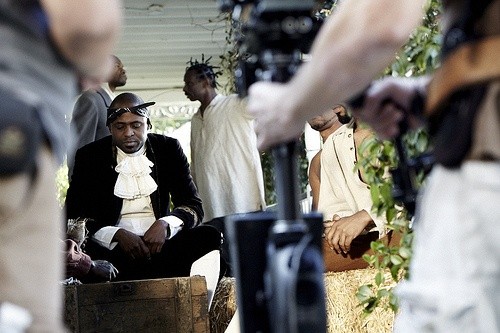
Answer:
[64,92,225,316]
[0,0,500,333]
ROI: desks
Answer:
[62,274,210,333]
